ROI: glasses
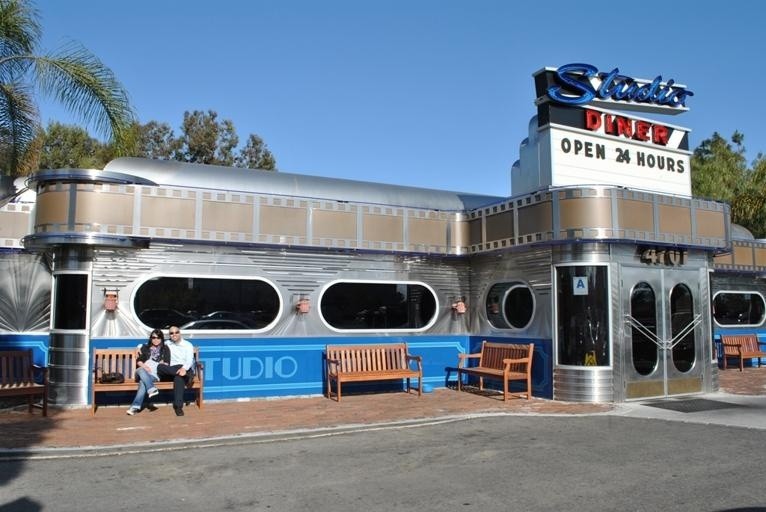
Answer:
[150,336,160,339]
[169,330,180,334]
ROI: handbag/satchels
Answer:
[98,371,125,384]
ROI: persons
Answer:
[125,329,170,416]
[133,324,195,416]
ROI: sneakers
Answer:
[174,405,184,416]
[186,370,196,389]
[148,387,159,398]
[126,407,137,416]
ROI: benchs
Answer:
[0,349,49,417]
[326,342,422,402]
[457,341,535,403]
[92,346,204,414]
[720,332,766,372]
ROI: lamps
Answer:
[451,301,465,314]
[296,298,311,313]
[104,293,117,311]
[488,303,499,313]
